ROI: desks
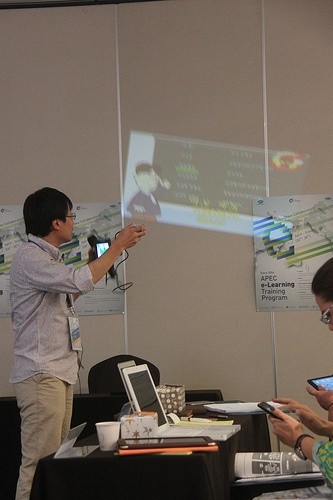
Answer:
[0,389,224,500]
[29,400,271,500]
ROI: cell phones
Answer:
[257,402,281,420]
[95,238,110,259]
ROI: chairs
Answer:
[88,354,160,394]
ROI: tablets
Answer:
[116,436,216,450]
[306,375,333,391]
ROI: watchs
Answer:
[294,434,315,460]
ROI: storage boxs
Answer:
[121,413,158,444]
[155,383,186,412]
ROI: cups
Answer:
[95,421,121,451]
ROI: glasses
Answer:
[320,305,333,324]
[66,213,76,220]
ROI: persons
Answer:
[268,257,333,500]
[9,187,147,500]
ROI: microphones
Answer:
[87,235,115,280]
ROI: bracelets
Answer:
[327,403,333,410]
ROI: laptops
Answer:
[122,363,241,441]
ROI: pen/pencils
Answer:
[208,414,227,419]
